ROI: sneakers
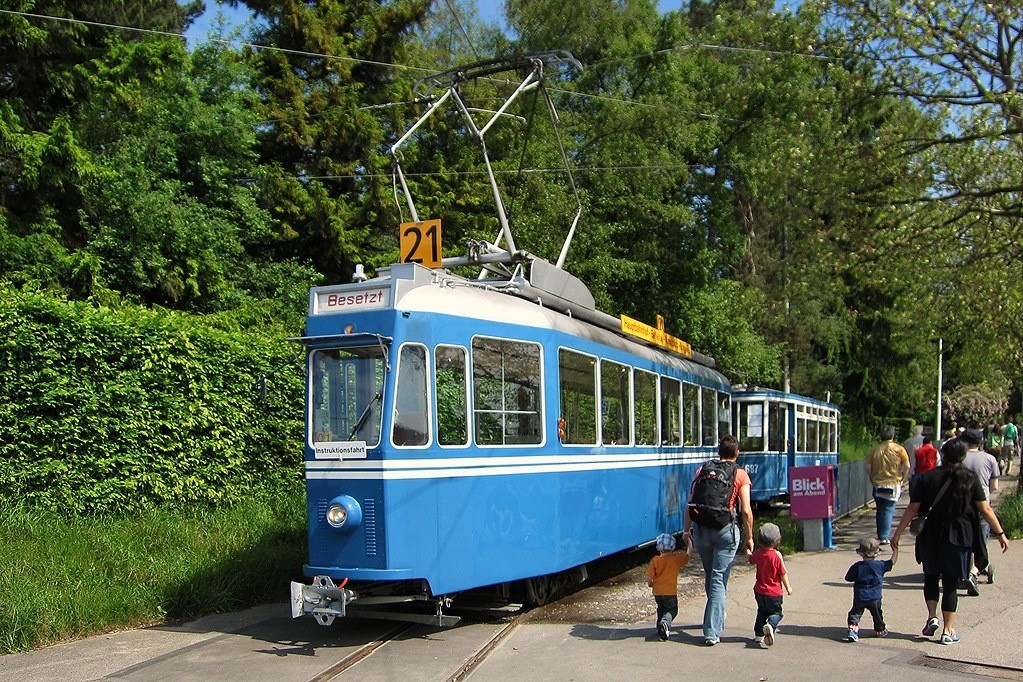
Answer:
[846,630,859,642]
[922,616,940,636]
[754,636,765,642]
[875,629,889,638]
[940,629,961,645]
[957,577,968,589]
[657,621,671,642]
[705,637,720,646]
[762,622,774,646]
[963,572,981,596]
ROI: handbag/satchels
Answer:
[909,516,927,537]
[873,483,902,502]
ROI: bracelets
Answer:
[683,529,691,534]
[997,532,1004,536]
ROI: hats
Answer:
[856,538,882,558]
[961,429,983,443]
[758,522,782,544]
[656,532,676,551]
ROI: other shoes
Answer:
[879,539,891,546]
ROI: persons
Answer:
[913,435,942,475]
[937,416,1023,594]
[745,522,793,647]
[866,423,910,545]
[843,537,898,641]
[682,434,754,646]
[902,424,925,479]
[891,438,1010,645]
[646,533,693,642]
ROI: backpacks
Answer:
[687,459,743,544]
[1003,422,1017,441]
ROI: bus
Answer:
[290,49,841,627]
